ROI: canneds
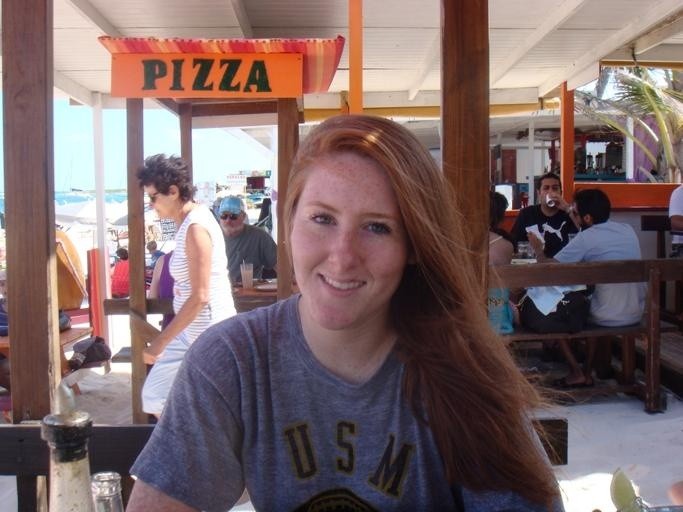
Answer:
[546,194,555,207]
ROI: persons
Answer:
[137,155,237,422]
[216,195,278,280]
[147,250,176,331]
[669,184,683,253]
[0,212,72,337]
[111,241,167,299]
[486,174,647,404]
[124,114,585,512]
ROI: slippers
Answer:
[553,377,584,388]
[585,379,594,387]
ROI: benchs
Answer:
[489,258,682,412]
[103,296,277,423]
[0,418,568,512]
[641,215,683,326]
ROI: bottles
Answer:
[90,471,125,512]
[40,410,97,511]
[238,263,253,288]
[35,474,48,511]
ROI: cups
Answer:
[516,242,535,259]
[545,194,554,207]
[524,223,545,246]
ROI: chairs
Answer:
[254,197,272,230]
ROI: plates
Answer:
[252,278,272,286]
[509,257,538,265]
[255,285,279,291]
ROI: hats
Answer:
[218,196,244,215]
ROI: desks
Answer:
[109,239,166,259]
[231,279,300,314]
[0,327,95,421]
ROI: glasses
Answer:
[148,192,159,203]
[219,213,239,220]
